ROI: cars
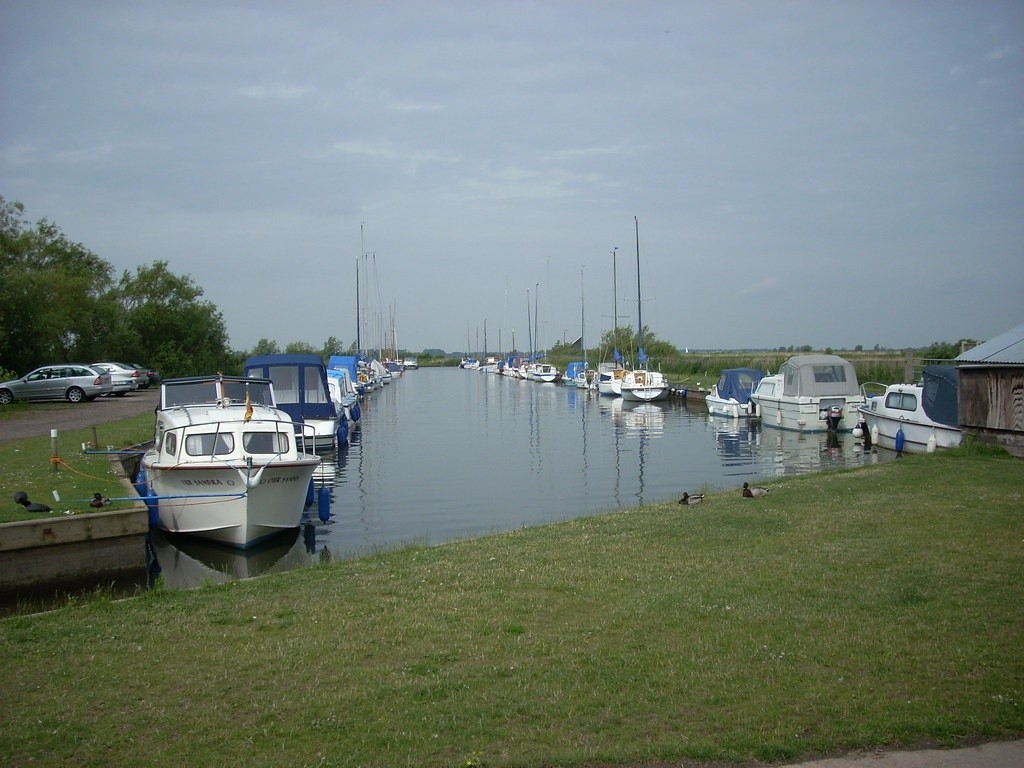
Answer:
[122,362,162,389]
[88,361,148,397]
[0,362,113,405]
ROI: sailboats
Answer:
[458,247,633,396]
[325,219,419,428]
[616,213,670,403]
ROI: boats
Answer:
[748,351,872,433]
[856,361,963,456]
[141,372,325,551]
[242,351,347,451]
[705,365,770,421]
